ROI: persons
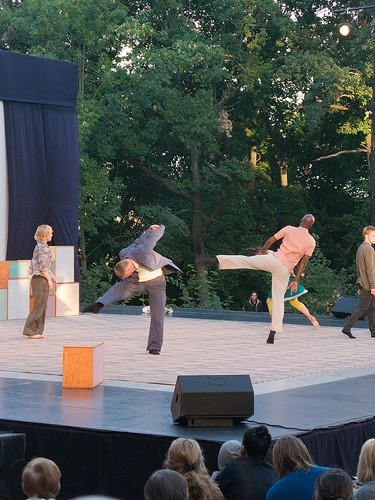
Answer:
[21,456,61,500]
[23,225,55,339]
[83,224,182,354]
[245,247,320,330]
[199,213,316,344]
[242,291,263,312]
[144,426,375,500]
[342,226,375,339]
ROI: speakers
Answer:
[170,374,255,425]
[0,430,27,500]
[331,296,365,321]
